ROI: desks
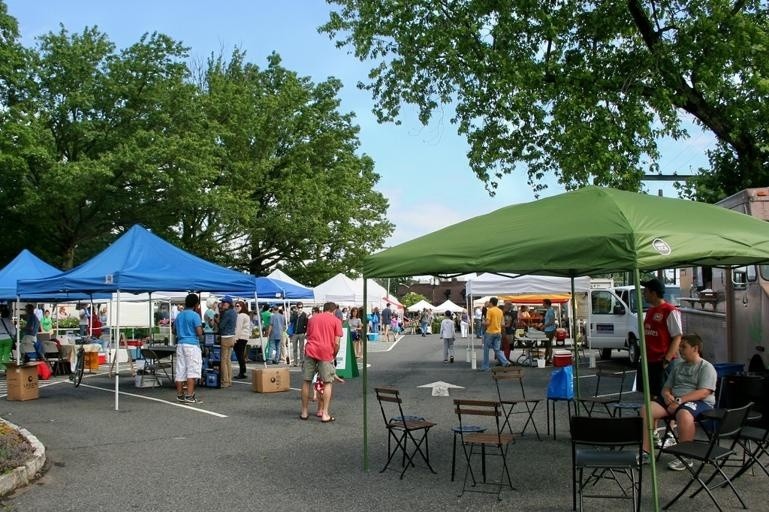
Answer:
[53,327,81,339]
[513,337,549,368]
[556,339,565,345]
[59,343,103,375]
[140,346,176,374]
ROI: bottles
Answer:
[526,327,528,337]
[524,326,527,335]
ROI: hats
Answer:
[219,295,232,303]
[640,278,665,292]
[207,297,221,303]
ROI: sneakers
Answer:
[636,452,657,466]
[668,456,693,471]
[184,392,204,404]
[657,433,677,449]
[177,392,185,402]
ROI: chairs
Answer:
[140,349,172,388]
[9,342,70,376]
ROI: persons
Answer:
[154,308,169,328]
[636,334,718,470]
[171,294,204,405]
[312,358,346,417]
[299,302,343,422]
[204,294,470,389]
[0,303,111,375]
[475,297,556,371]
[638,279,683,449]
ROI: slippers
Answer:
[321,417,335,422]
[300,414,309,420]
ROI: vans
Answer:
[586,283,687,359]
[678,187,769,392]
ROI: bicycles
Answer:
[68,338,85,387]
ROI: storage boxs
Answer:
[555,327,566,339]
[1,361,43,401]
[252,367,290,393]
[198,332,258,387]
[553,354,573,366]
[367,333,379,341]
[83,340,144,370]
[135,375,162,387]
[37,333,51,342]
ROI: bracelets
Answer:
[663,359,670,364]
[675,398,681,405]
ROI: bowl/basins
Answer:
[519,334,524,337]
